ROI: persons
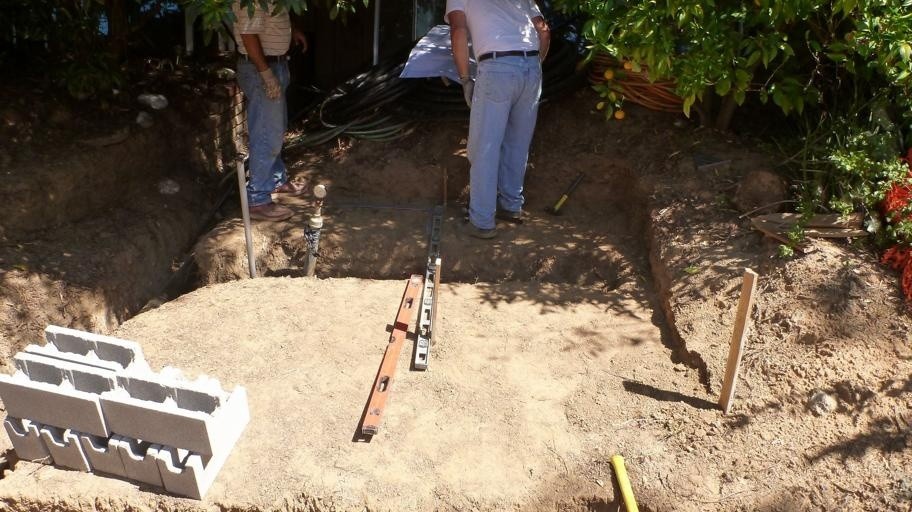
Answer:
[443,1,553,239]
[231,1,309,224]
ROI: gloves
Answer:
[461,74,475,107]
[259,67,282,99]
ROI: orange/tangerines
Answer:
[604,70,614,79]
[615,110,625,120]
[623,62,632,70]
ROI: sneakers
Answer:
[270,180,307,199]
[496,201,522,219]
[464,220,498,238]
[247,201,295,222]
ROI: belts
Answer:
[478,49,540,61]
[243,50,289,63]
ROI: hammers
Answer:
[465,205,523,225]
[543,171,585,217]
